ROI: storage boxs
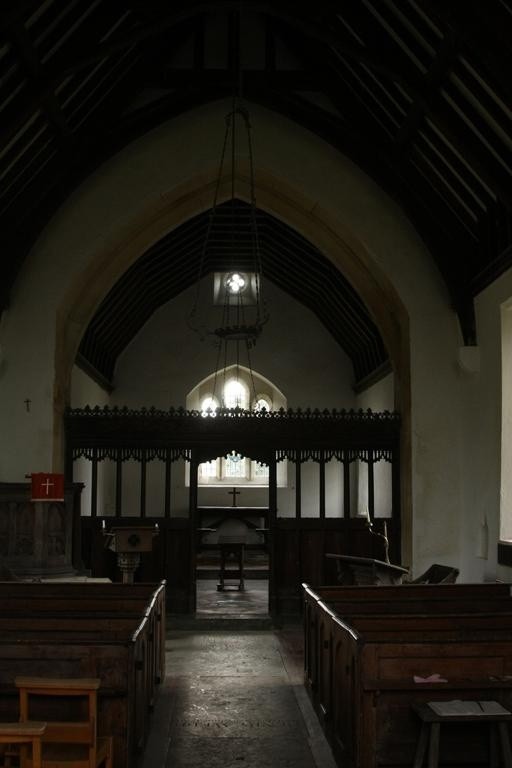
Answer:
[0,578,168,768]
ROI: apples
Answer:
[384,522,388,540]
[102,520,105,527]
[366,505,369,521]
[155,522,158,527]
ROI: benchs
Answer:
[295,577,510,766]
[0,578,168,768]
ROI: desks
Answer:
[216,534,247,593]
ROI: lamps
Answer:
[195,283,276,419]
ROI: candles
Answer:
[102,520,105,527]
[366,505,369,521]
[155,522,158,527]
[384,522,388,540]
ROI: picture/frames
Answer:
[216,534,247,593]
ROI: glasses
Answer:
[195,283,276,419]
